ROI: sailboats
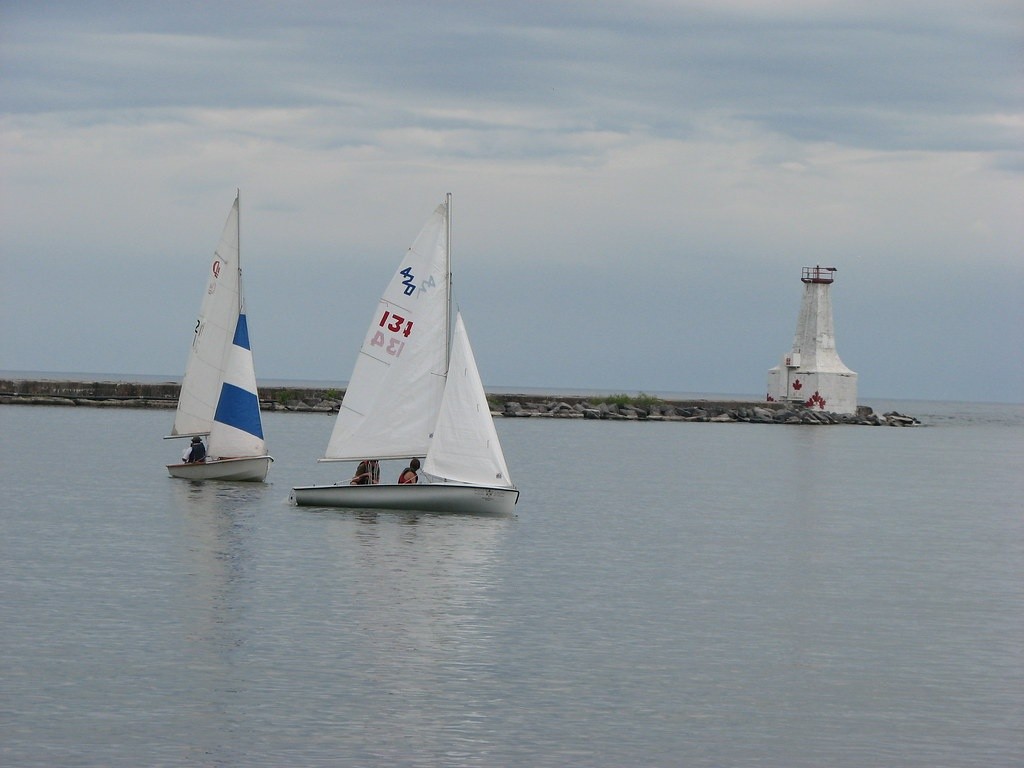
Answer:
[290,192,519,515]
[162,188,276,482]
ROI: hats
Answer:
[191,435,202,441]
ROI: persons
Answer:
[182,435,206,463]
[398,459,421,484]
[350,460,380,485]
